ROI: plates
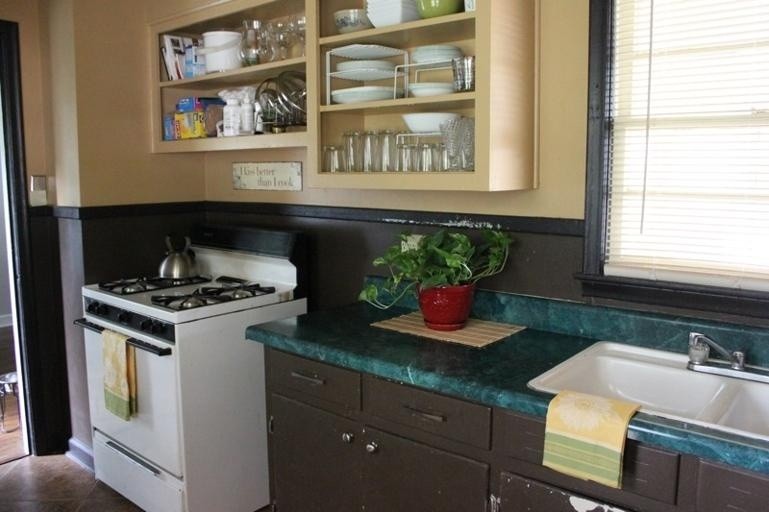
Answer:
[327,43,463,103]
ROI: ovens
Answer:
[75,314,271,512]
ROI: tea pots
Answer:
[158,233,197,278]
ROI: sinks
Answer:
[706,375,769,442]
[523,339,728,421]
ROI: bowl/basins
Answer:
[332,0,463,35]
[401,111,463,133]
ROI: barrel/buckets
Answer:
[193,30,246,73]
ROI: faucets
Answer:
[687,327,769,384]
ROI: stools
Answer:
[0,372,20,433]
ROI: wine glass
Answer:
[238,17,306,65]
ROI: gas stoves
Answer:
[80,273,291,343]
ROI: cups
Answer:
[321,117,474,173]
[451,57,475,93]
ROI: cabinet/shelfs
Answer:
[306,0,538,192]
[147,0,307,153]
[263,342,497,512]
[494,404,769,512]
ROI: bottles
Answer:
[222,93,264,137]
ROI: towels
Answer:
[541,390,642,490]
[371,310,527,348]
[101,328,138,422]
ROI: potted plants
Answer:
[356,223,514,330]
